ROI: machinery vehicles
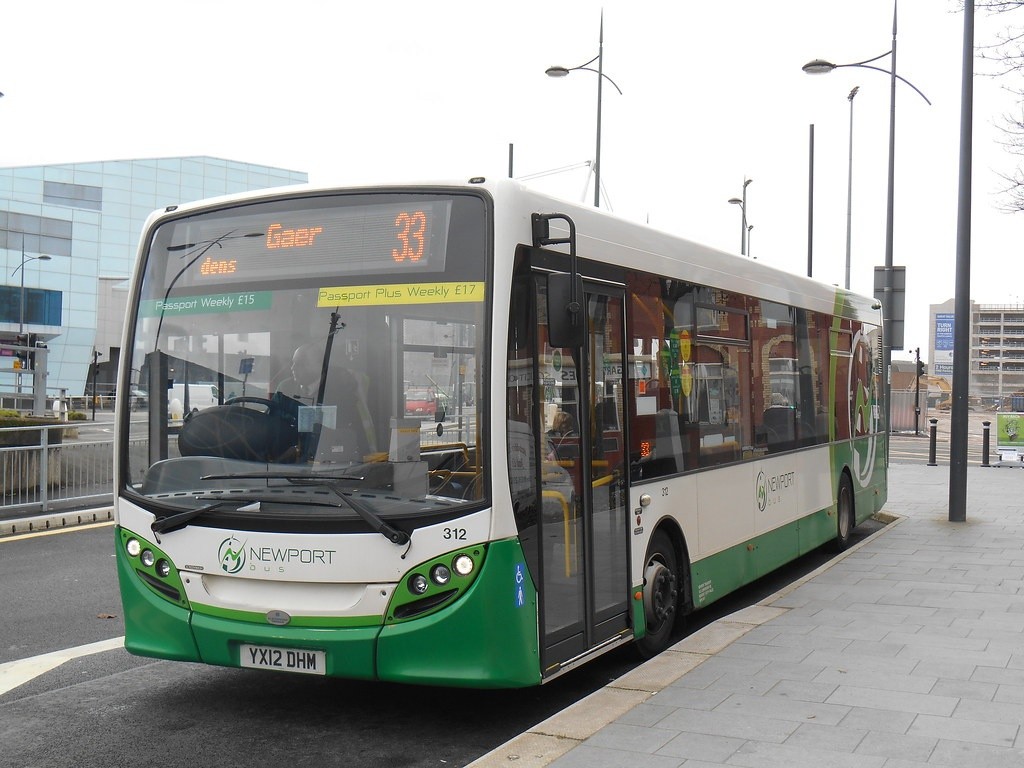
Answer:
[904,374,980,412]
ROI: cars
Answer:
[435,392,452,415]
[109,389,150,413]
[404,389,439,418]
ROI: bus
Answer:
[110,172,894,693]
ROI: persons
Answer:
[545,411,579,459]
[263,344,350,462]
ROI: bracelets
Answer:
[294,445,300,456]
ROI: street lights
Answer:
[544,5,623,212]
[802,0,933,472]
[727,178,753,256]
[13,235,52,334]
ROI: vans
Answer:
[168,385,225,419]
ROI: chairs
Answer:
[269,362,837,500]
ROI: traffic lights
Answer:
[15,332,29,369]
[31,333,47,370]
[918,361,925,376]
